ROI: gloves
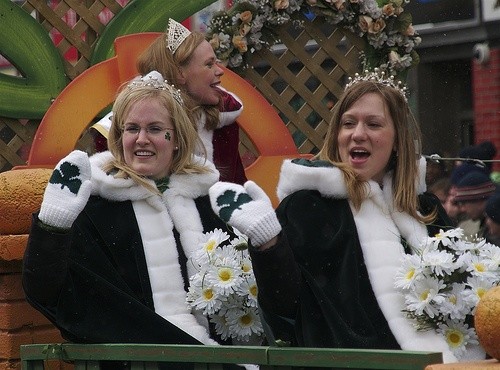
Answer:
[209,180,282,247]
[38,150,92,228]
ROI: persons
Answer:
[425,140,500,247]
[22,71,269,370]
[73,17,248,186]
[210,68,493,370]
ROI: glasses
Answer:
[123,126,174,137]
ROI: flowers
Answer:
[202,1,418,76]
[187,229,267,340]
[402,226,499,356]
